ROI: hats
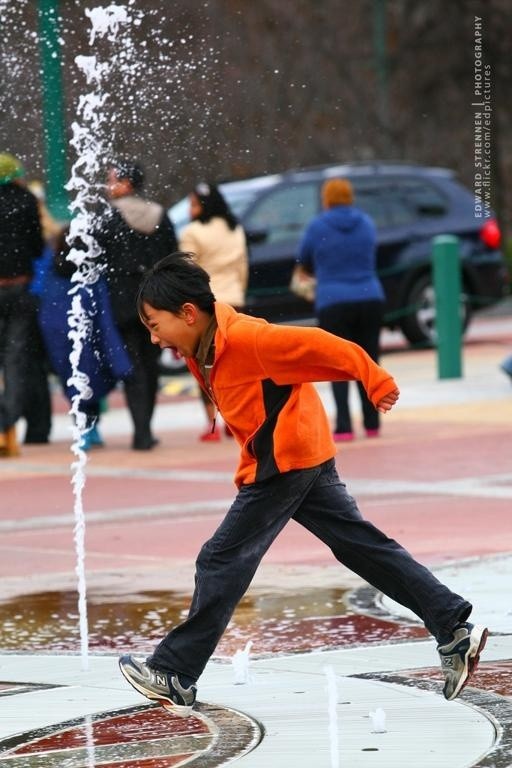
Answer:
[321,177,354,208]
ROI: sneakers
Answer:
[332,429,380,441]
[436,621,488,701]
[199,424,233,442]
[81,428,103,450]
[119,654,197,717]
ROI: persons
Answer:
[298,178,388,441]
[118,251,487,718]
[0,153,249,456]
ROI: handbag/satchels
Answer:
[288,262,317,304]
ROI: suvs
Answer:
[158,154,509,380]
